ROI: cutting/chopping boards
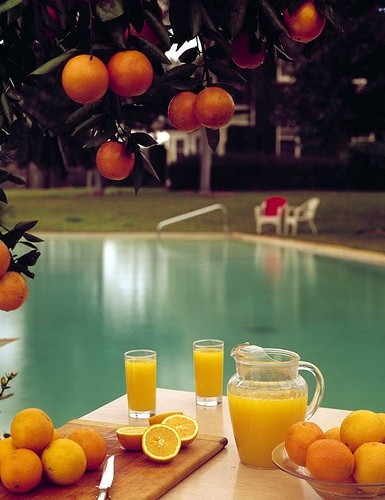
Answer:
[0,419,228,500]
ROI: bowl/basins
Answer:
[271,441,385,499]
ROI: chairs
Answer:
[283,196,320,236]
[253,196,288,236]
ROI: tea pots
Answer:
[226,341,325,471]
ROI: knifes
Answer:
[95,454,116,500]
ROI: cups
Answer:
[124,349,157,420]
[193,339,225,407]
